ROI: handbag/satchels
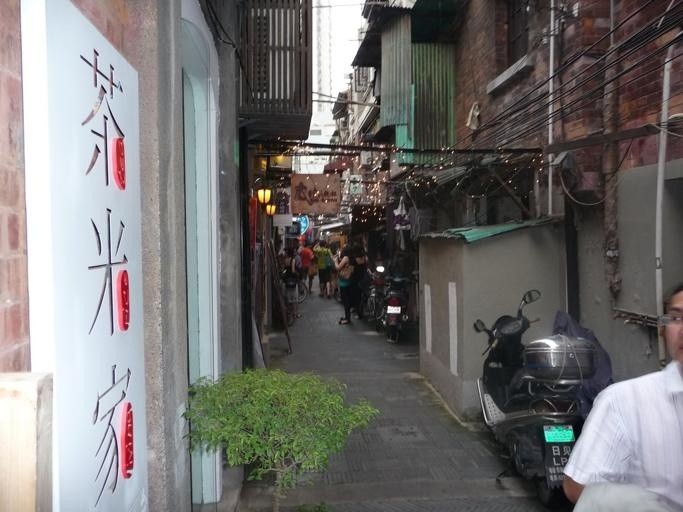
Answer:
[338,264,354,279]
[325,255,332,268]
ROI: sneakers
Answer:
[340,317,350,324]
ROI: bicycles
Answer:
[360,268,386,323]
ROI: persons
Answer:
[281,239,368,324]
[562,283,683,512]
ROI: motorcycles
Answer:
[376,264,411,346]
[473,289,598,506]
